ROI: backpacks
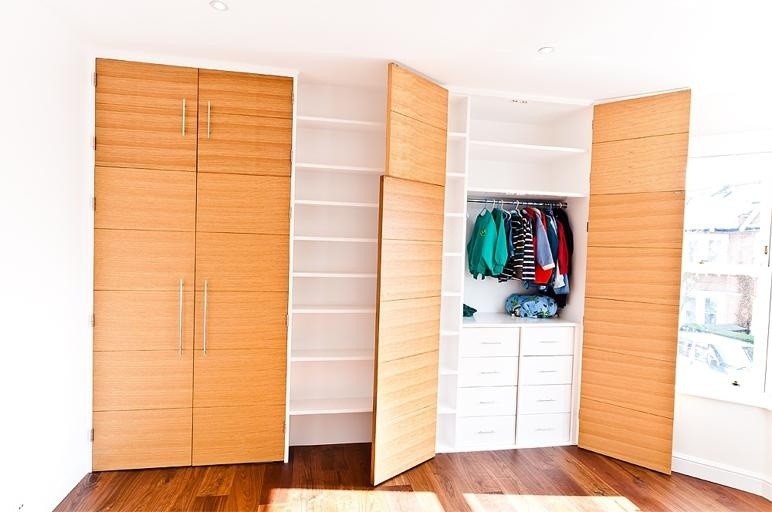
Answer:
[505,293,558,319]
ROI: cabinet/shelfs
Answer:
[458,326,575,452]
[466,87,592,197]
[91,57,292,474]
[284,73,469,464]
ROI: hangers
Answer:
[480,199,562,215]
